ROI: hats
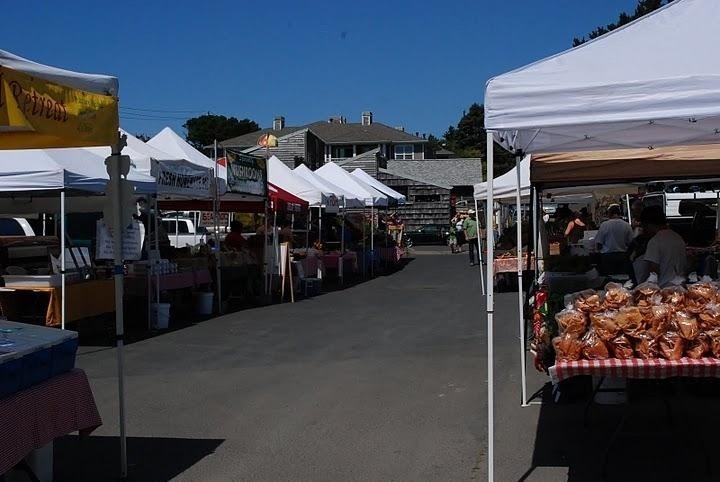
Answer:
[468,209,476,214]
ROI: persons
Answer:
[225,221,247,246]
[564,215,589,244]
[464,209,485,266]
[593,200,636,274]
[640,205,689,277]
[448,209,487,254]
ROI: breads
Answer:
[552,282,720,360]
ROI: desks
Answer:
[0,367,87,481]
[553,355,720,482]
[126,269,211,302]
